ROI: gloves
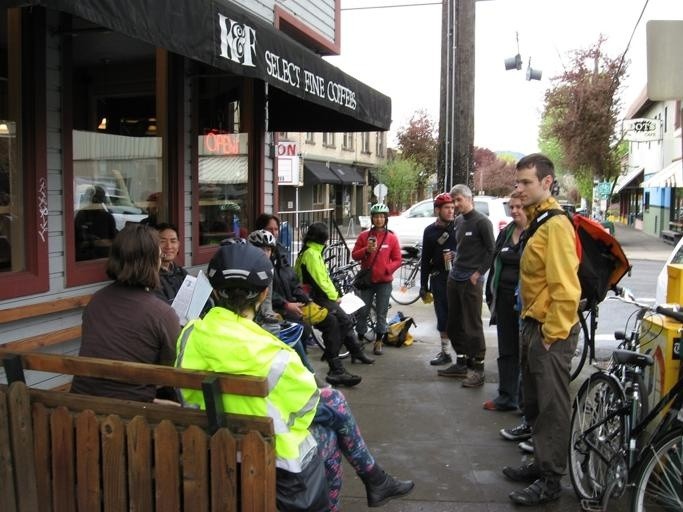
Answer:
[418,286,433,304]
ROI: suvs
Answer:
[381,194,514,257]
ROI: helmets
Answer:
[371,204,389,214]
[206,243,274,290]
[247,230,277,248]
[259,322,303,348]
[434,192,454,206]
[219,238,247,247]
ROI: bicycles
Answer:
[589,287,682,498]
[388,247,422,304]
[568,311,588,381]
[568,328,683,512]
[309,241,375,358]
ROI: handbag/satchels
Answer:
[516,210,617,305]
[352,229,387,288]
[382,314,414,346]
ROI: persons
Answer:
[248,229,333,389]
[438,183,496,388]
[173,243,415,512]
[68,224,182,466]
[482,190,527,415]
[416,193,458,365]
[155,222,213,330]
[352,204,401,355]
[294,222,376,365]
[256,214,363,386]
[73,187,119,261]
[501,230,537,453]
[138,193,158,230]
[503,154,581,505]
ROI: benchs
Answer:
[0,350,278,512]
[0,291,91,393]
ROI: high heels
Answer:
[349,346,375,364]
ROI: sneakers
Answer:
[430,352,453,365]
[320,350,340,360]
[500,425,533,440]
[437,365,468,378]
[365,469,415,505]
[503,462,541,480]
[520,437,540,454]
[462,366,484,388]
[481,391,517,413]
[374,342,386,353]
[508,477,563,504]
[326,359,362,386]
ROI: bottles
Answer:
[368,235,377,247]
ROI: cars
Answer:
[654,235,683,306]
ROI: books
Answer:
[171,269,213,327]
[338,290,366,315]
